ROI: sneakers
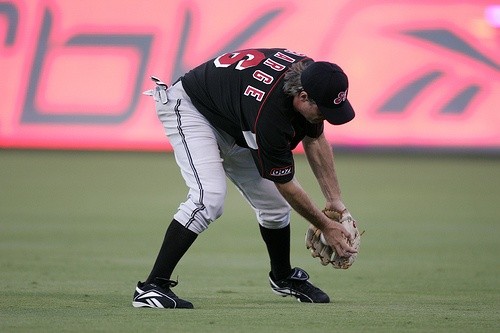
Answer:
[269,267,330,303]
[132,275,194,309]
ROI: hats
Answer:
[301,61,355,125]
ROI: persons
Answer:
[131,48,358,310]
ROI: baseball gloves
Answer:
[304,208,360,269]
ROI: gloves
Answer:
[142,76,169,104]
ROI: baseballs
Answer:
[320,232,328,245]
